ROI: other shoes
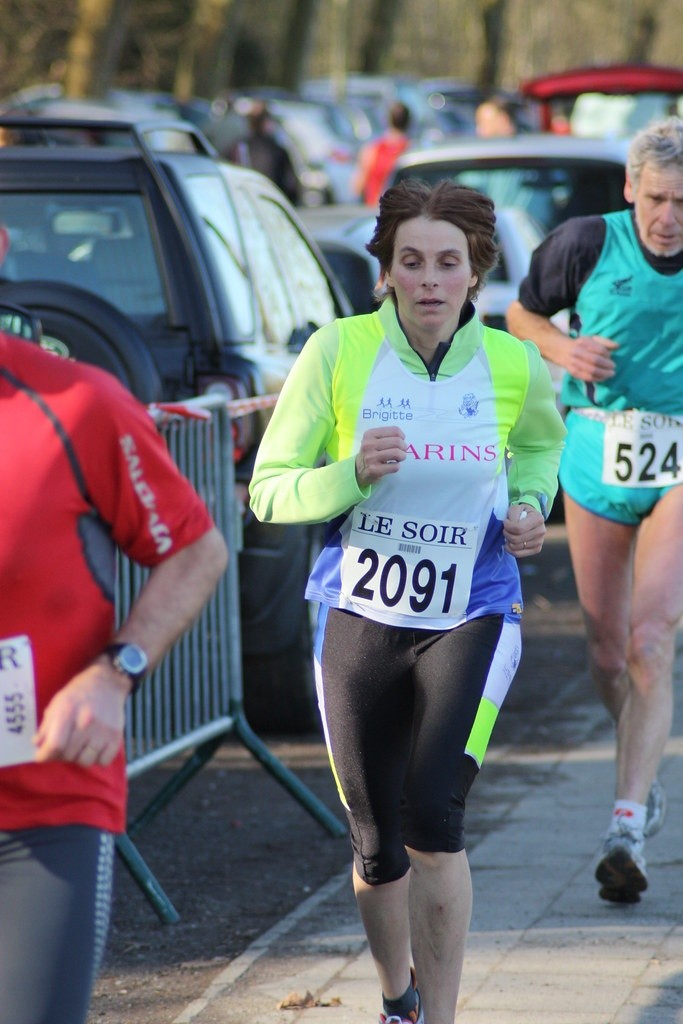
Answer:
[379,966,425,1024]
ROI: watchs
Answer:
[102,641,149,693]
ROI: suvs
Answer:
[1,110,359,739]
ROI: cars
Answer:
[0,62,682,411]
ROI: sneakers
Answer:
[642,780,666,838]
[593,833,649,903]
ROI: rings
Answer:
[523,542,527,548]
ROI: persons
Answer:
[475,96,525,136]
[0,223,230,1024]
[352,102,412,207]
[247,178,568,1024]
[505,115,683,907]
[230,109,303,208]
